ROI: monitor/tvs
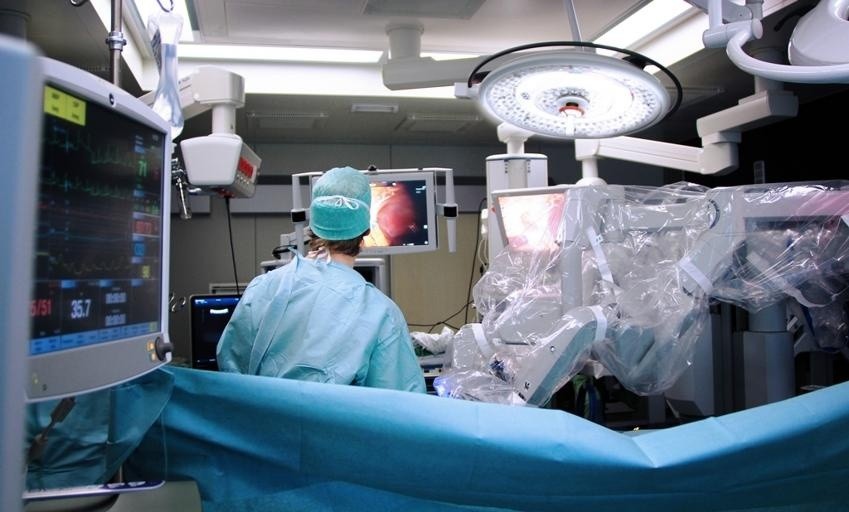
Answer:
[188,292,242,371]
[311,172,440,257]
[25,55,173,404]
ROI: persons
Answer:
[216,166,427,395]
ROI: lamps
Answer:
[381,19,683,139]
